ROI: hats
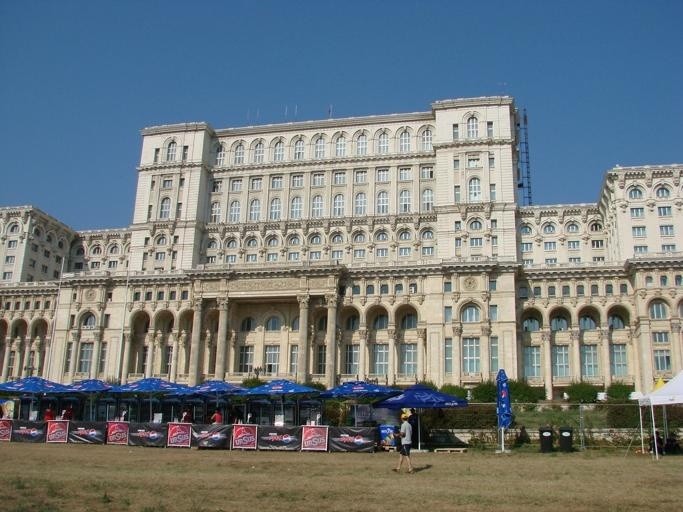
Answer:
[401,414,409,419]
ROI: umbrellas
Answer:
[496,369,513,452]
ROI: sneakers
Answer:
[405,467,416,474]
[391,468,400,472]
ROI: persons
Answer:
[665,433,681,453]
[649,431,666,455]
[392,413,414,474]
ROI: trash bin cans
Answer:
[539,427,553,453]
[559,427,572,452]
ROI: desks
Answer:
[300,418,378,453]
[105,419,172,449]
[166,418,305,452]
[0,418,109,446]
